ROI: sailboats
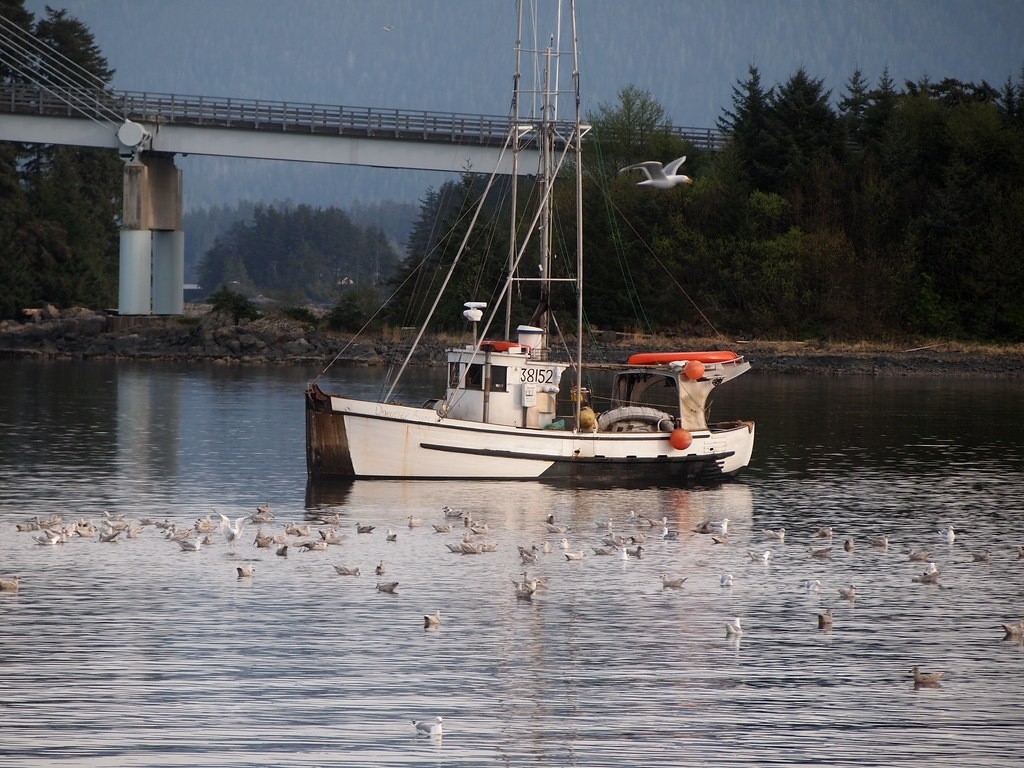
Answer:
[304,0,756,486]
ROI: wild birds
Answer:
[619,157,695,189]
[0,497,1023,741]
[382,25,395,34]
